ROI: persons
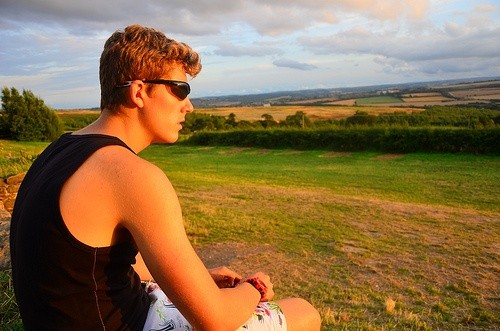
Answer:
[9,22,325,330]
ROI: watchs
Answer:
[235,277,268,305]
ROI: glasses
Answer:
[116,79,191,100]
